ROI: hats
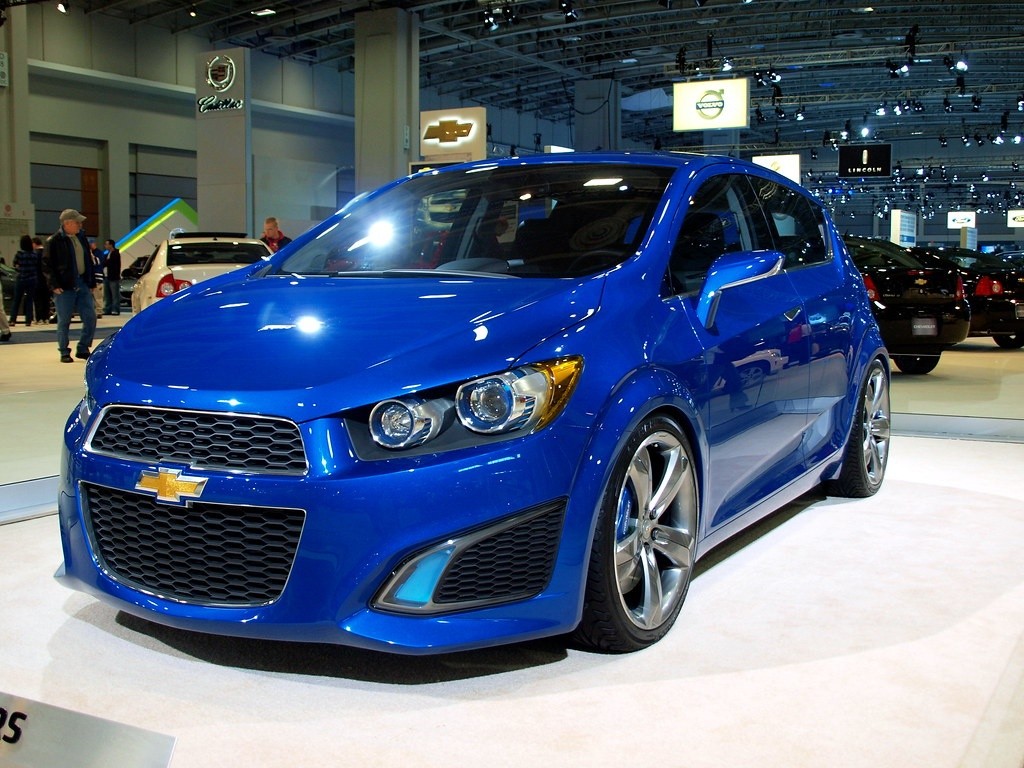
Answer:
[87,238,96,246]
[57,209,87,223]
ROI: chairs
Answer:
[665,212,725,294]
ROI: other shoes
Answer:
[60,354,74,363]
[76,350,91,360]
[100,310,120,315]
[7,315,59,326]
[0,332,11,343]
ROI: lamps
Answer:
[483,0,1024,220]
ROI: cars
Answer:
[0,263,95,324]
[128,232,272,317]
[118,255,149,304]
[57,150,893,658]
[927,244,1024,351]
[835,233,971,374]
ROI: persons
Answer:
[88,239,106,320]
[1,284,12,344]
[7,234,38,327]
[258,216,293,255]
[32,237,51,325]
[42,208,98,363]
[488,216,510,251]
[100,240,122,316]
